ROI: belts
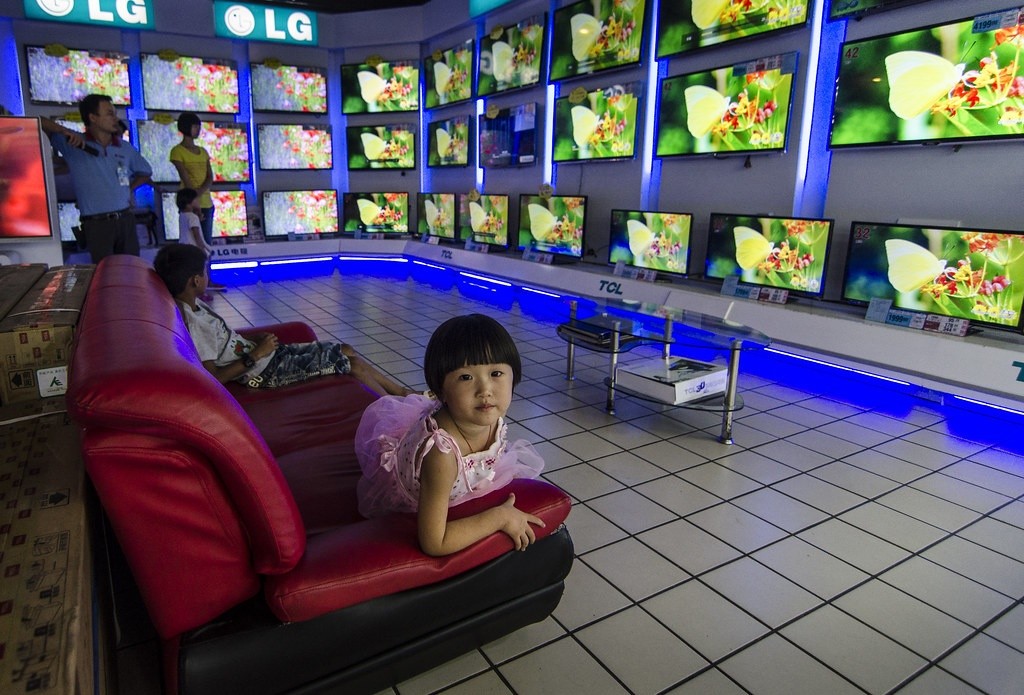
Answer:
[82,208,132,222]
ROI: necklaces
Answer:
[452,417,492,453]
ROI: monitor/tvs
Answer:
[418,39,474,238]
[704,212,834,299]
[826,0,1024,152]
[340,59,421,235]
[136,51,250,241]
[457,10,549,248]
[517,1,648,258]
[24,44,134,247]
[0,116,54,242]
[841,221,1024,329]
[652,0,809,160]
[608,209,695,275]
[257,123,334,170]
[345,125,416,170]
[250,63,339,237]
[550,81,642,164]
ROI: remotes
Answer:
[65,135,99,156]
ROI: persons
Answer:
[177,189,214,301]
[39,94,153,266]
[169,111,227,290]
[154,243,425,398]
[354,313,546,556]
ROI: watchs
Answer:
[242,353,255,368]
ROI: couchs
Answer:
[64,254,571,695]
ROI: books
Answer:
[558,313,643,345]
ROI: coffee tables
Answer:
[554,294,773,446]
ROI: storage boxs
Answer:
[616,355,728,405]
[0,264,117,695]
